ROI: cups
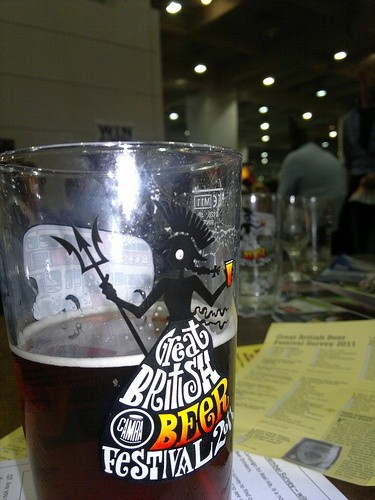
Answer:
[1,140,238,500]
[231,192,284,315]
[280,197,333,283]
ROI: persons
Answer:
[341,51,374,186]
[272,115,349,236]
[332,159,374,254]
